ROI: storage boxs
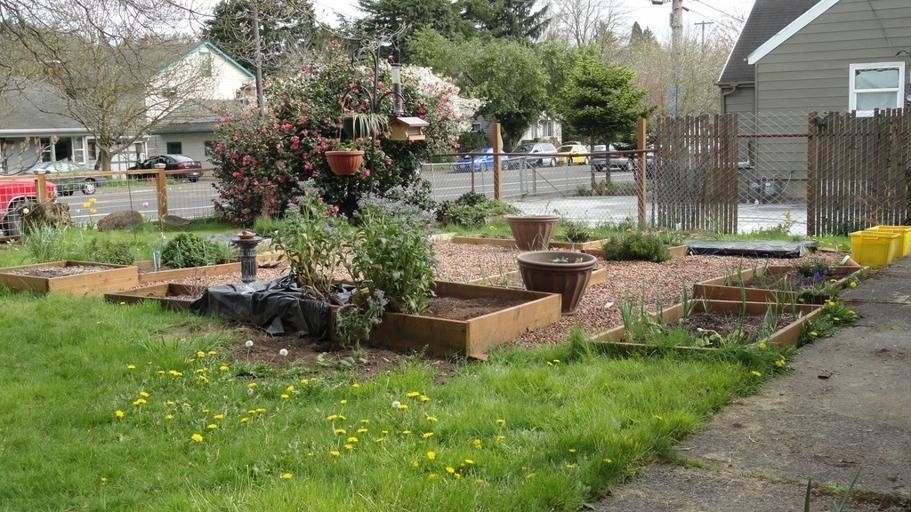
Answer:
[848,225,911,266]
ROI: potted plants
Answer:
[325,142,365,176]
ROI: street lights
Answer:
[143,134,149,160]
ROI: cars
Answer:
[0,160,107,242]
[452,140,654,172]
[126,154,204,182]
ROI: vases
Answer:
[517,250,597,316]
[506,215,560,251]
